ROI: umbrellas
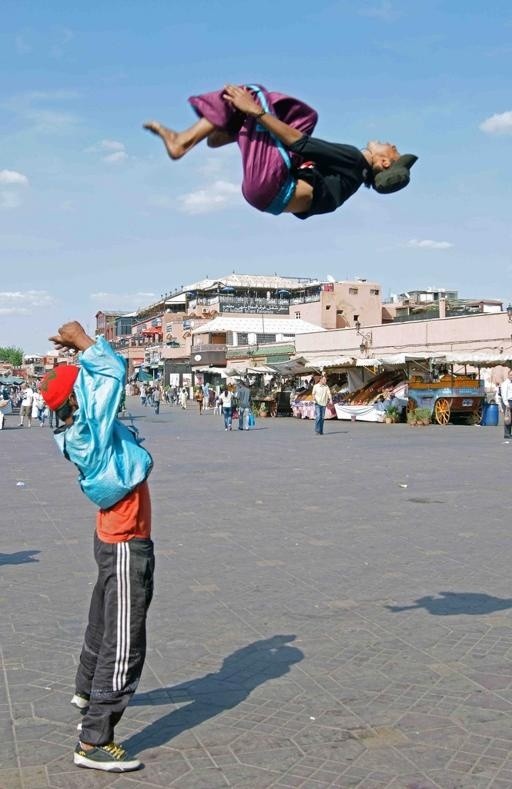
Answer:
[222,286,236,295]
[274,290,291,300]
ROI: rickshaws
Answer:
[269,390,294,418]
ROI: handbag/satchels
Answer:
[248,411,255,426]
[504,407,512,426]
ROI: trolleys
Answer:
[404,358,487,425]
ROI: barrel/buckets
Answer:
[485,403,499,426]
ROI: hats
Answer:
[38,365,80,412]
[369,151,419,194]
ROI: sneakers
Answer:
[70,687,90,711]
[72,740,142,773]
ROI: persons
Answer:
[311,376,333,434]
[142,82,417,221]
[35,320,156,772]
[140,380,312,432]
[501,368,512,445]
[0,376,60,430]
